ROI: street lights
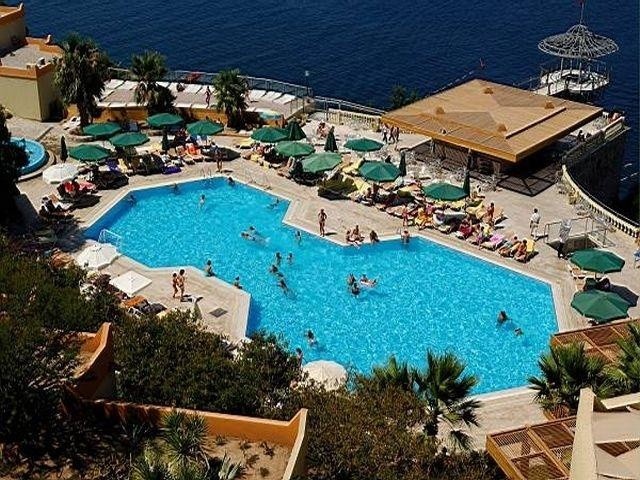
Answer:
[304,70,310,98]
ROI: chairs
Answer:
[238,120,539,263]
[38,133,242,230]
[120,294,149,309]
[128,303,167,319]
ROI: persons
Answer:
[402,184,542,264]
[56,118,224,202]
[499,311,506,323]
[173,260,243,298]
[177,81,183,92]
[317,208,327,237]
[243,89,250,103]
[296,348,304,368]
[348,272,368,295]
[239,225,302,289]
[346,224,379,250]
[203,85,211,107]
[242,114,409,209]
